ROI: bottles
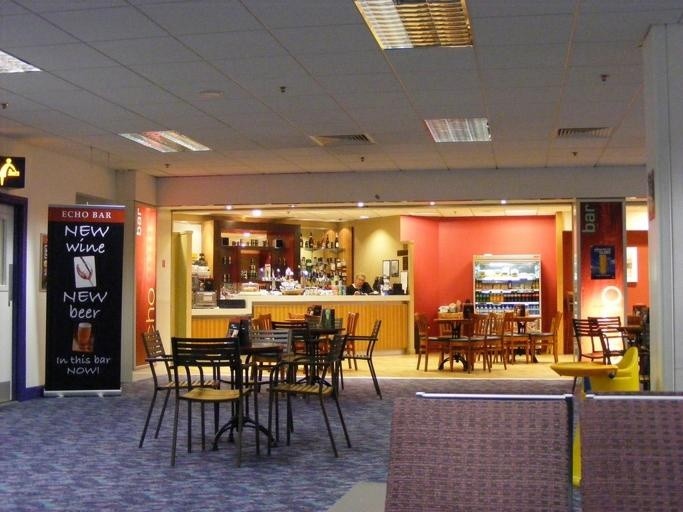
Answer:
[599,255,608,275]
[300,233,347,285]
[222,239,287,283]
[475,280,539,315]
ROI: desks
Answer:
[551,359,617,395]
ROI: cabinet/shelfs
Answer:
[300,225,355,287]
[201,219,302,292]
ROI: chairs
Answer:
[413,311,562,375]
[385,395,683,512]
[571,304,650,395]
[140,312,383,468]
[573,347,640,486]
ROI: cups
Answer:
[77,323,91,349]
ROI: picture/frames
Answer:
[590,245,615,280]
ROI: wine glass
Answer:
[77,261,94,286]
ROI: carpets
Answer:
[1,375,584,510]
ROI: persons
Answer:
[346,273,373,295]
